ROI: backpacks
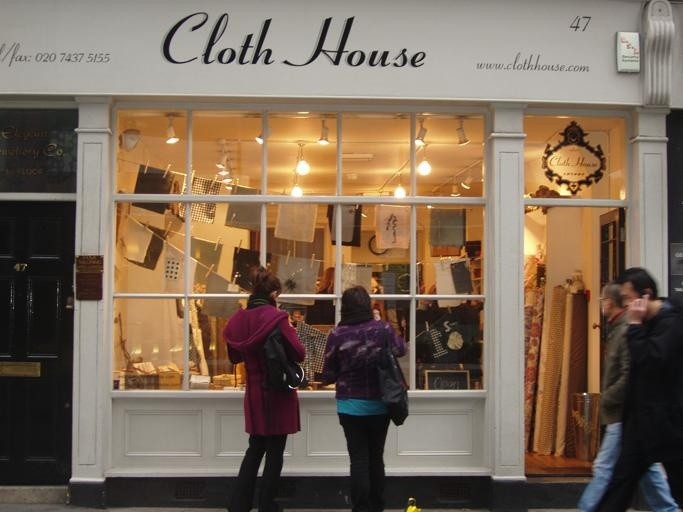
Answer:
[259,330,306,397]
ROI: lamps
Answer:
[165,114,180,145]
[416,119,428,144]
[461,169,473,190]
[290,172,301,196]
[417,151,431,176]
[457,118,470,147]
[450,177,462,197]
[257,126,272,145]
[214,137,238,190]
[296,143,309,174]
[393,174,408,198]
[317,119,329,146]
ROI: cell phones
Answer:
[640,287,647,300]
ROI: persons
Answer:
[594,266,682,511]
[220,266,306,512]
[575,280,682,510]
[320,284,408,511]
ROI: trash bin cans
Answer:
[569,393,601,460]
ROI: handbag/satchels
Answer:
[371,361,409,427]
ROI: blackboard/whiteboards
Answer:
[424,369,470,390]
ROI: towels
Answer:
[114,204,473,383]
[225,184,263,233]
[131,163,174,215]
[171,170,222,225]
